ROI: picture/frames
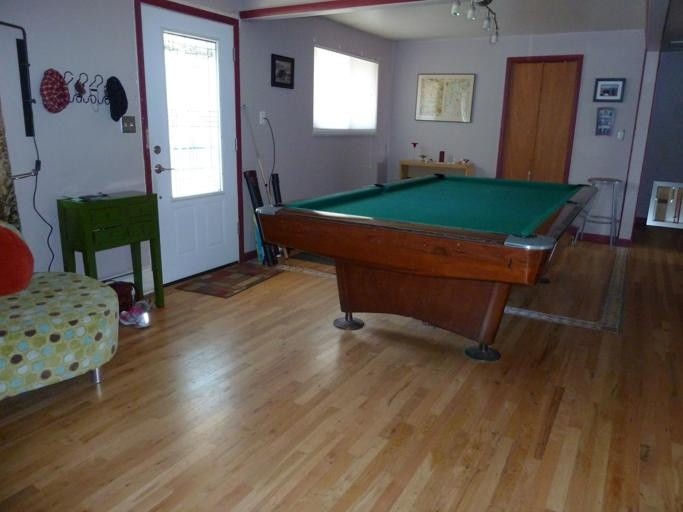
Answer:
[595,106,617,136]
[270,53,295,89]
[592,78,626,102]
[415,73,475,123]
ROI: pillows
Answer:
[0,220,34,298]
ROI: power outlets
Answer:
[259,111,271,125]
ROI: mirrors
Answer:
[646,180,683,229]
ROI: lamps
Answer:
[450,0,500,44]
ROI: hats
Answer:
[39,69,70,114]
[106,77,127,122]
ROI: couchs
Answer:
[0,220,119,401]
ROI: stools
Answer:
[573,177,623,249]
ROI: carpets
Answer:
[175,262,284,298]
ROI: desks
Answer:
[57,190,166,309]
[255,173,598,362]
[399,158,473,180]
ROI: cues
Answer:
[242,104,288,259]
